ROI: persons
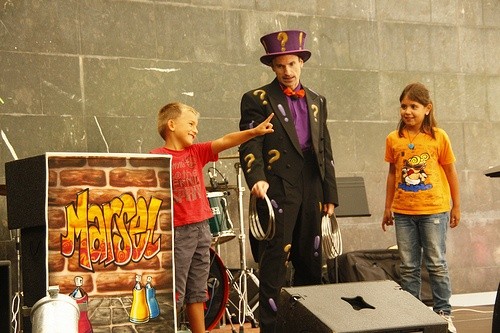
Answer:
[147,102,274,333]
[381,82,461,333]
[238,31,339,333]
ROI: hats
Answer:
[260,29,312,67]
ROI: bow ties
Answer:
[283,87,305,100]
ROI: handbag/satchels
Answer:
[327,248,437,306]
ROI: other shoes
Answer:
[439,314,457,333]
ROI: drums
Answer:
[183,248,228,331]
[205,189,237,247]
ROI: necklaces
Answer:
[406,127,421,148]
